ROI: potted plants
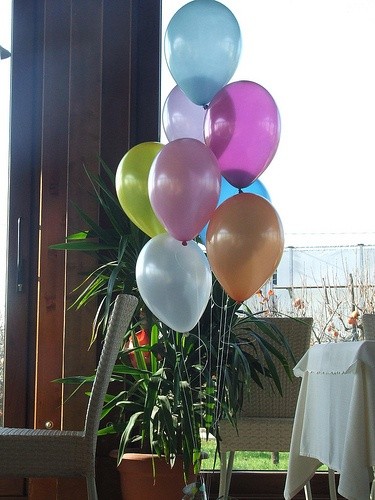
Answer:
[46,155,320,500]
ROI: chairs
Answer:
[0,293,138,500]
[217,317,313,500]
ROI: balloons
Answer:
[147,138,221,246]
[206,192,284,303]
[115,142,167,237]
[164,0,242,110]
[200,175,272,246]
[161,85,209,145]
[135,233,212,336]
[202,80,282,193]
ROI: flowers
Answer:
[254,288,367,347]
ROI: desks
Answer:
[283,341,375,500]
